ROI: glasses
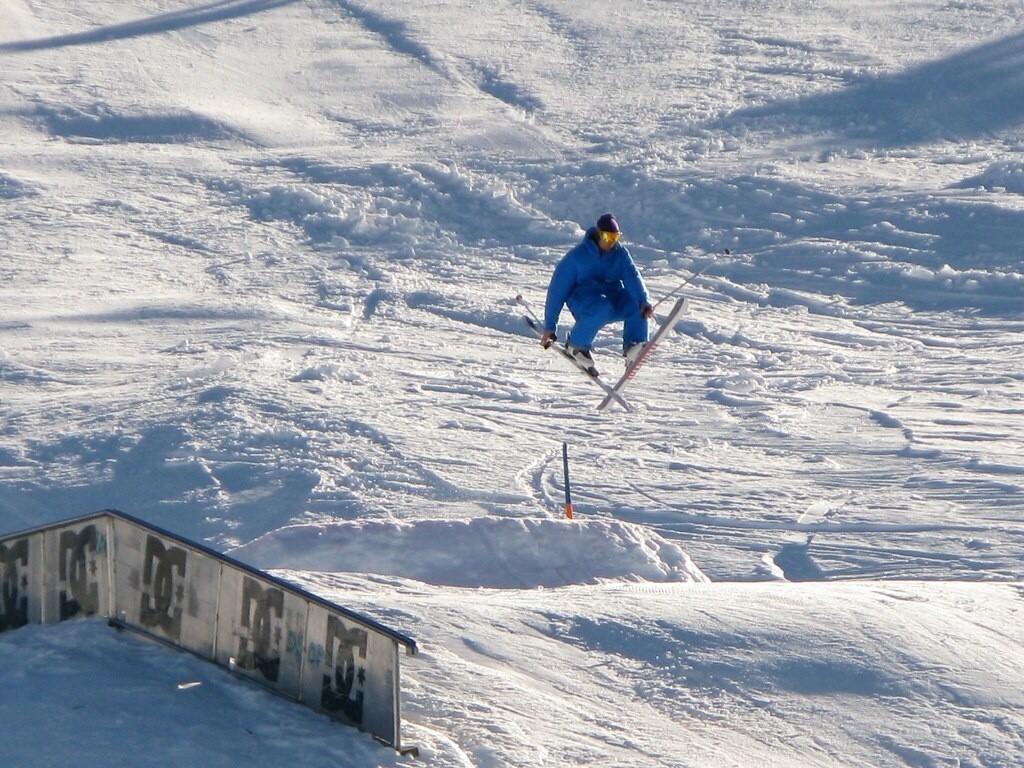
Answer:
[599,231,623,243]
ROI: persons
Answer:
[540,213,658,368]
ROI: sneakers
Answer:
[566,342,595,368]
[624,342,646,368]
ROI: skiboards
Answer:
[522,294,688,414]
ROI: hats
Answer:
[596,213,620,233]
